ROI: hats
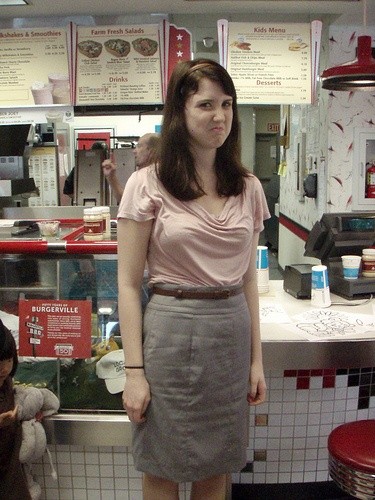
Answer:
[95,347,128,395]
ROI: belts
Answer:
[148,286,244,301]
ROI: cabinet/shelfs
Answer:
[0,217,149,447]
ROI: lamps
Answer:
[319,0,375,93]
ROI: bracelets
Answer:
[123,365,144,369]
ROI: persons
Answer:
[0,319,44,496]
[102,133,162,205]
[117,58,271,500]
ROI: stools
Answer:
[327,419,375,500]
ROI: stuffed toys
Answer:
[12,386,60,496]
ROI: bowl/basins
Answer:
[38,221,59,237]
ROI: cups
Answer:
[311,265,331,309]
[255,245,270,296]
[341,255,361,280]
